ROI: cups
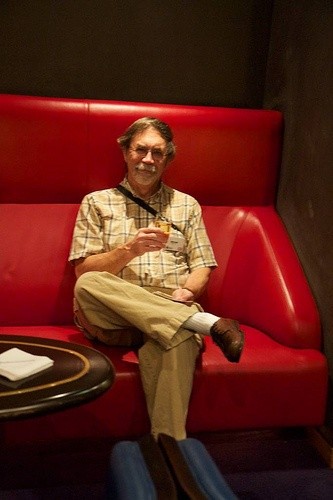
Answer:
[155,212,173,246]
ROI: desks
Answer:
[0,333,117,421]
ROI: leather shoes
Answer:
[209,318,244,363]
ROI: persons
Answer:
[66,115,244,442]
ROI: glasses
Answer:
[133,147,167,160]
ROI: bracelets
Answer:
[181,287,197,298]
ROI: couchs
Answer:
[0,94,327,444]
